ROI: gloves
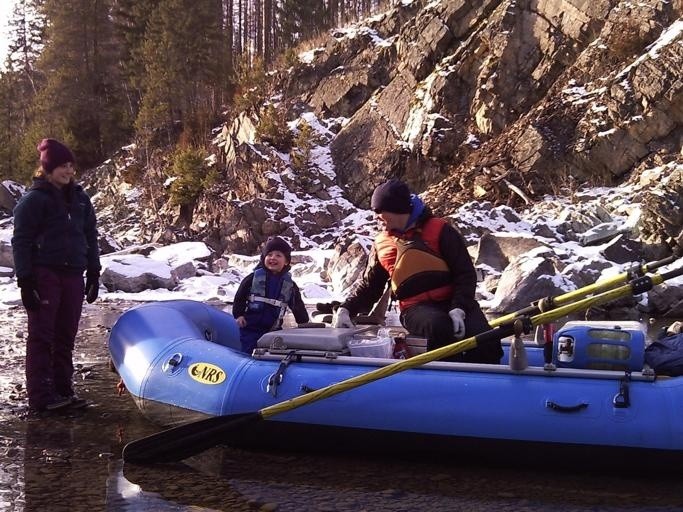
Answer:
[332,307,356,328]
[85,272,100,303]
[17,274,41,309]
[449,308,467,340]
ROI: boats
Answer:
[108,298,683,482]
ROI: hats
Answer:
[37,138,74,174]
[263,236,291,258]
[371,179,412,215]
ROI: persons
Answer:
[11,138,103,410]
[233,237,311,355]
[332,179,504,364]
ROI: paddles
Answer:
[122,267,683,464]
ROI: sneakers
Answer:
[39,393,71,410]
[61,394,88,410]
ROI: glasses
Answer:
[372,209,387,215]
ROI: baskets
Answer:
[349,338,393,358]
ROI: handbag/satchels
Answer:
[644,332,683,375]
